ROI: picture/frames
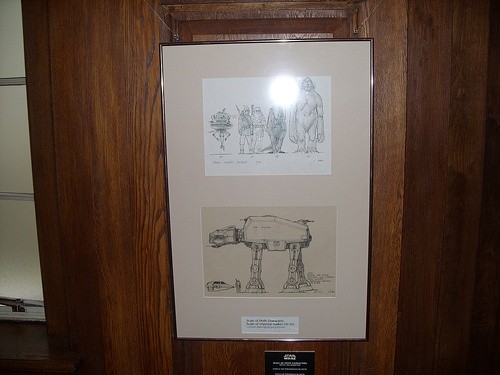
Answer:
[159,38,375,343]
